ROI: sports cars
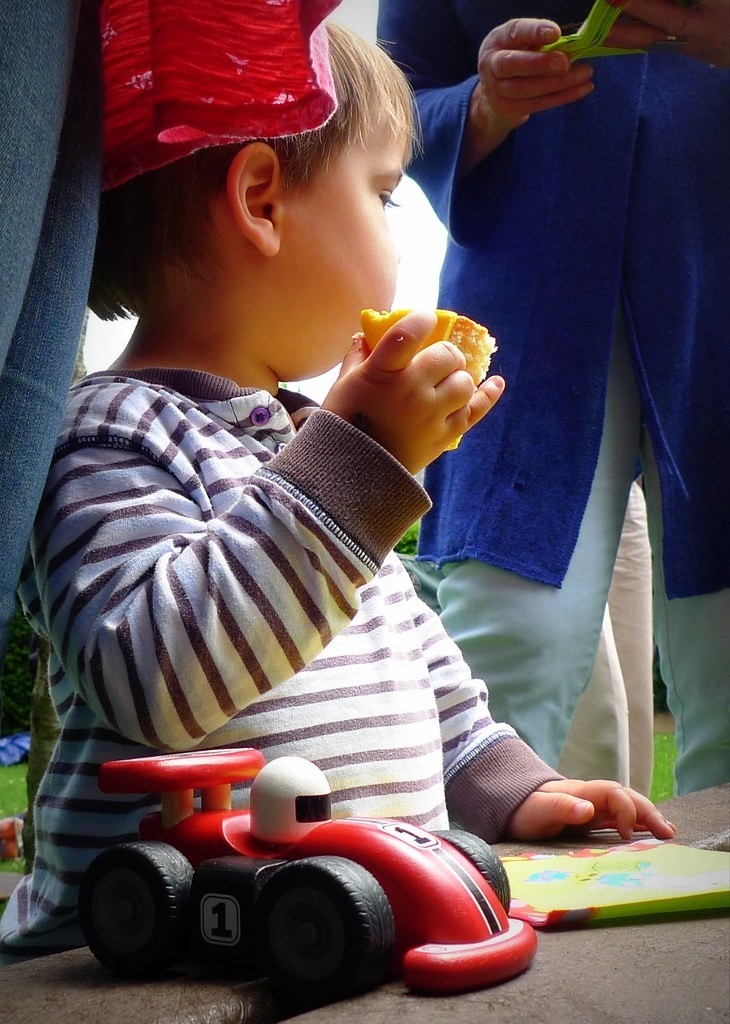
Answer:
[76,747,541,1013]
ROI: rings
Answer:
[668,35,676,40]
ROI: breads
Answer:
[358,308,496,450]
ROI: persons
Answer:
[0,0,345,620]
[376,0,730,791]
[0,22,676,964]
[554,470,655,799]
